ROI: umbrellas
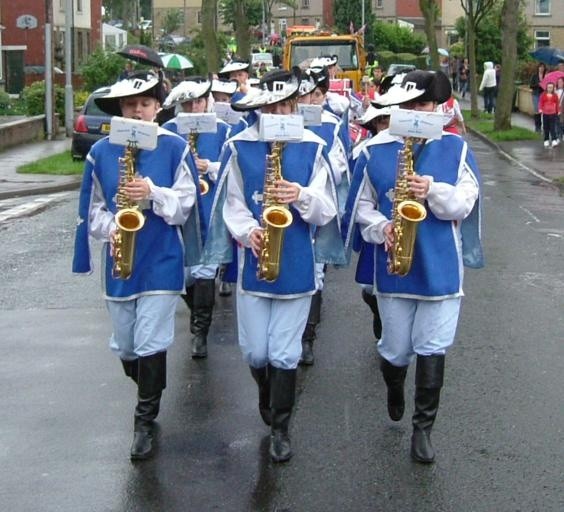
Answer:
[526,46,564,71]
[114,43,165,69]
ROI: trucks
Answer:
[283,27,364,95]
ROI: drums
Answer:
[322,76,351,109]
[349,122,371,154]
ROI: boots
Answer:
[120,350,166,461]
[410,352,445,466]
[382,359,408,420]
[361,286,383,341]
[180,276,233,359]
[250,362,296,464]
[298,289,322,367]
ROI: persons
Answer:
[530,60,564,148]
[158,20,501,464]
[69,72,205,461]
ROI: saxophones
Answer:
[256,141,292,283]
[185,130,208,195]
[384,134,426,277]
[109,147,144,281]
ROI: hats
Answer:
[355,63,451,132]
[93,54,338,117]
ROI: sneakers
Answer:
[543,138,560,147]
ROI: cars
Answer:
[71,84,124,160]
